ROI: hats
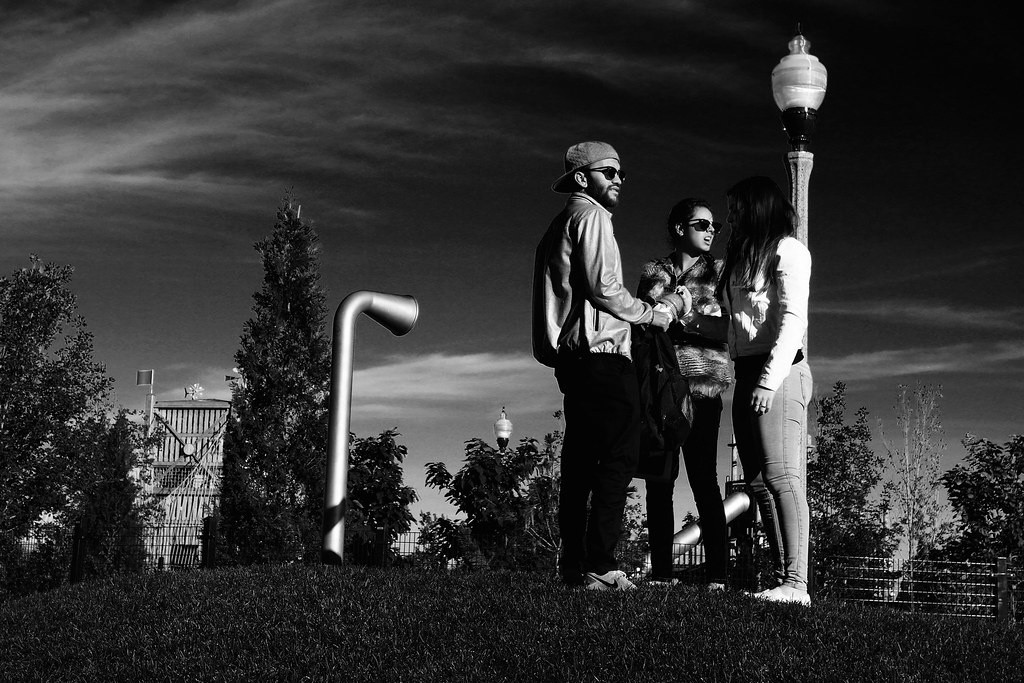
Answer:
[551,141,619,194]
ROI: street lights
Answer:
[770,25,829,243]
[494,405,514,460]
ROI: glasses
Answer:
[684,219,722,233]
[590,166,627,182]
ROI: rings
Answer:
[753,403,759,407]
[760,405,765,408]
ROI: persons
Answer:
[530,139,677,597]
[631,196,738,595]
[708,174,818,607]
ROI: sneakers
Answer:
[584,570,637,592]
[743,586,811,607]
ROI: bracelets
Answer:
[645,309,654,328]
